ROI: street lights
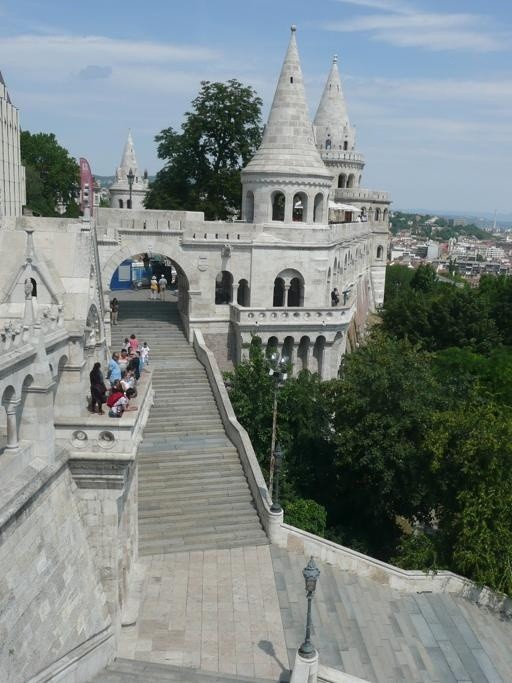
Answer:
[264,349,290,499]
[268,439,283,514]
[296,554,322,659]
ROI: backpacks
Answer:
[106,391,127,407]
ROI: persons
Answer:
[158,274,167,300]
[331,287,340,305]
[110,296,119,325]
[150,275,158,300]
[89,332,151,417]
